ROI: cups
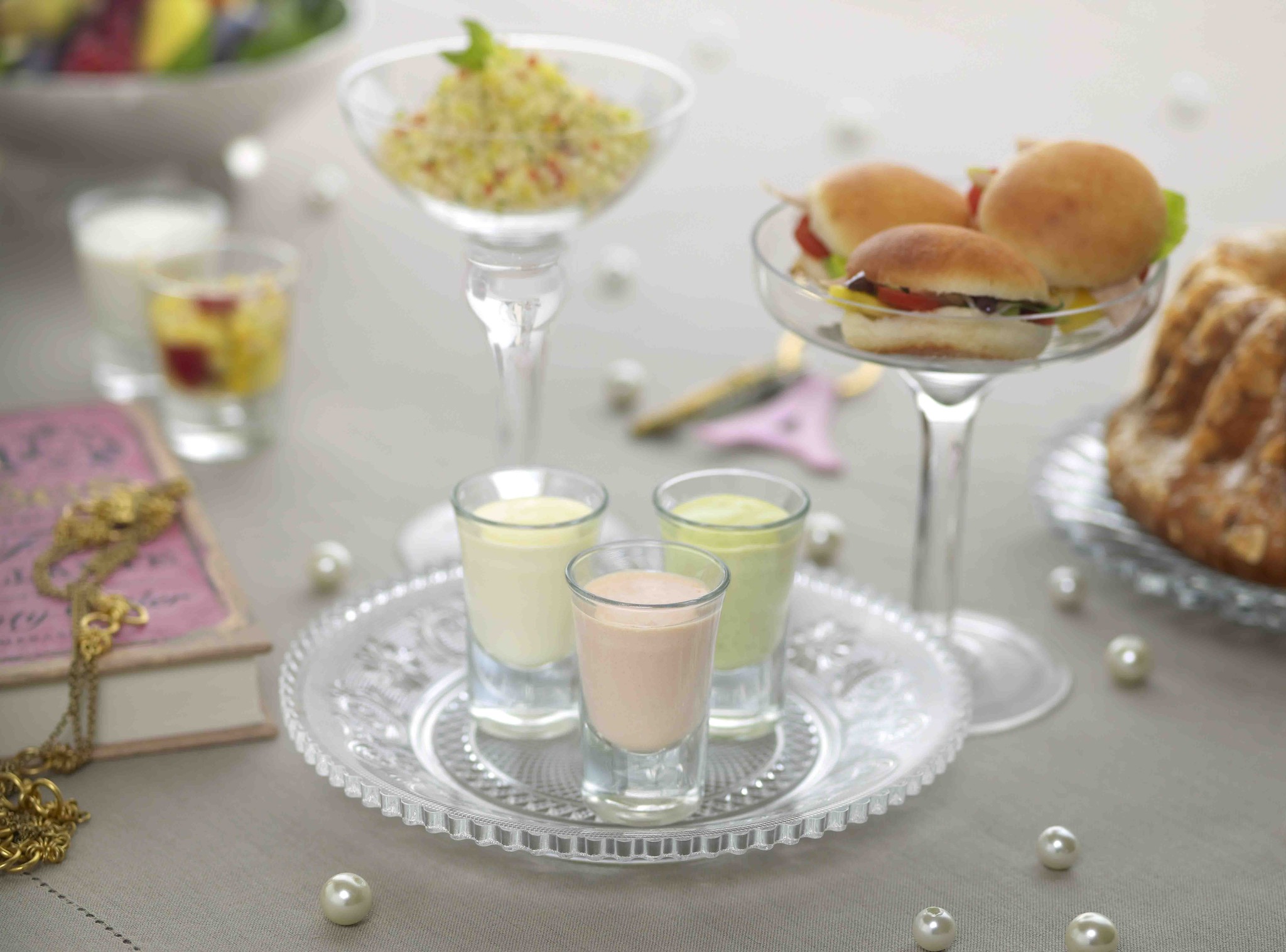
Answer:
[569,539,727,820]
[450,459,607,732]
[651,468,811,738]
[151,239,296,465]
[70,179,226,402]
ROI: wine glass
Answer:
[748,200,1168,735]
[337,36,692,573]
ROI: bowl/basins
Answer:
[3,31,353,182]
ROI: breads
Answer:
[1110,224,1286,586]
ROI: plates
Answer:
[278,553,970,872]
[1029,402,1283,638]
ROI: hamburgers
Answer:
[767,160,971,290]
[803,224,1070,359]
[971,140,1165,284]
